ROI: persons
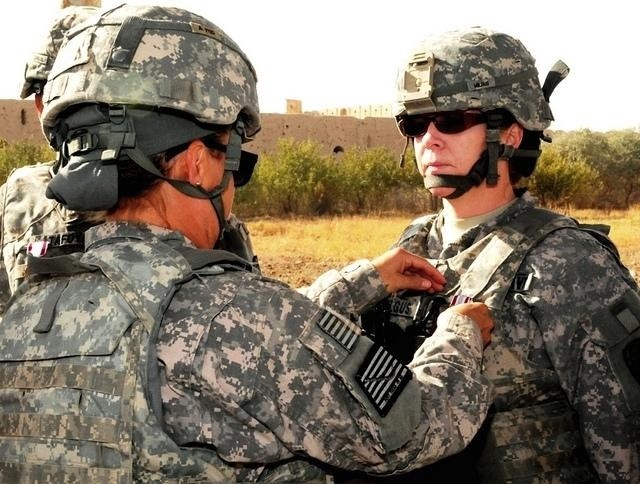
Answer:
[334,23,640,483]
[0,0,492,484]
[0,4,261,310]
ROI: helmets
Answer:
[41,6,260,145]
[393,27,552,132]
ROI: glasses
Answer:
[165,139,257,187]
[403,113,514,136]
